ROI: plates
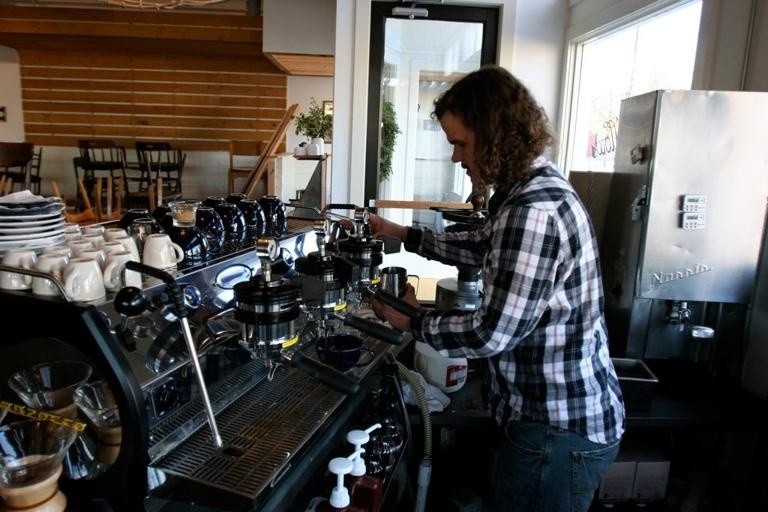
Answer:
[0,196,67,260]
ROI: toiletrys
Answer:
[313,447,368,512]
[346,422,384,512]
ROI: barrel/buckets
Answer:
[414,340,469,394]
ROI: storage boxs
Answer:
[597,442,672,500]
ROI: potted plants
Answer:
[292,97,333,155]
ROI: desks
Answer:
[402,363,767,511]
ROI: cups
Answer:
[259,194,287,236]
[0,248,36,292]
[316,334,377,374]
[62,257,106,302]
[33,254,71,297]
[198,191,266,248]
[45,222,140,270]
[103,250,145,293]
[381,266,420,302]
[142,232,184,269]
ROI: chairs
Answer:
[228,141,270,199]
[0,140,187,226]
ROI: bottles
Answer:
[117,200,211,262]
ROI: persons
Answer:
[368,66,628,512]
